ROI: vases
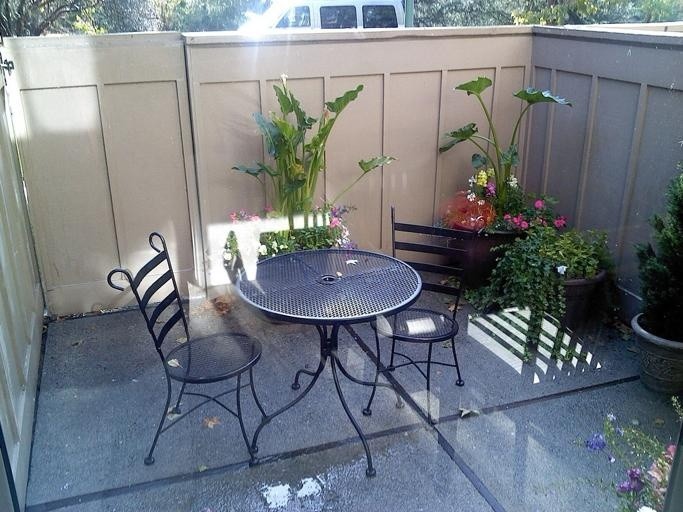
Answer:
[561,270,608,332]
[444,226,529,286]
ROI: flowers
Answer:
[224,72,398,259]
[444,220,618,369]
[582,394,683,512]
[439,72,577,228]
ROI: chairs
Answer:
[358,200,495,420]
[106,229,272,471]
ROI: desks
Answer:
[230,249,425,483]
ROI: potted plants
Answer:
[630,155,683,397]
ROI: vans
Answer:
[235,1,405,33]
